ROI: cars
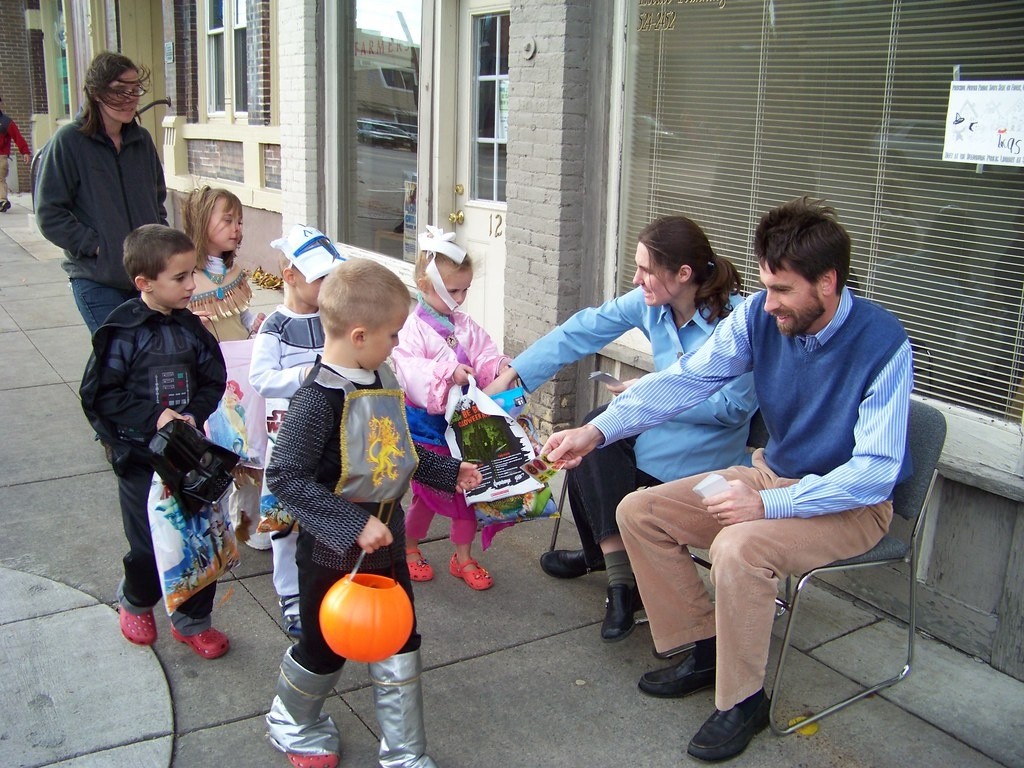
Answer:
[356,119,417,152]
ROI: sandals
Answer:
[405,549,433,581]
[171,622,229,659]
[278,594,303,638]
[119,605,157,645]
[286,751,340,768]
[449,553,493,590]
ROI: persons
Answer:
[0,97,31,213]
[263,258,482,768]
[387,224,518,591]
[78,224,231,658]
[33,52,166,462]
[178,185,271,550]
[249,222,346,634]
[482,215,760,642]
[535,195,912,762]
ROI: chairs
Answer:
[549,398,946,736]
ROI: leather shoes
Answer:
[540,550,606,579]
[687,688,771,763]
[601,572,642,643]
[638,652,716,697]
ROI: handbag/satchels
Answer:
[204,340,269,469]
[149,472,240,617]
[148,419,240,522]
[444,374,546,507]
[474,387,560,529]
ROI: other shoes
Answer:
[0,201,11,212]
[244,530,272,550]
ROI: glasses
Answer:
[109,86,147,98]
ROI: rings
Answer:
[717,512,720,520]
